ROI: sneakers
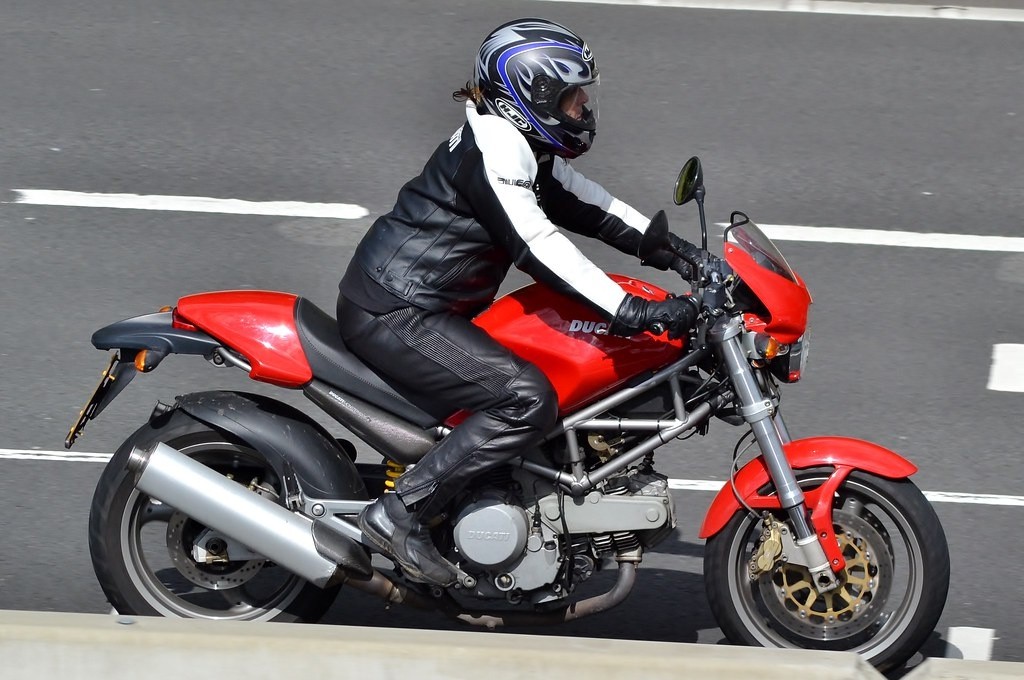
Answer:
[358,498,459,587]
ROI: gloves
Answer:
[646,233,719,287]
[607,294,693,339]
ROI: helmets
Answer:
[473,18,603,160]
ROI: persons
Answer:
[335,17,722,589]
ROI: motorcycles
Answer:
[64,154,954,677]
